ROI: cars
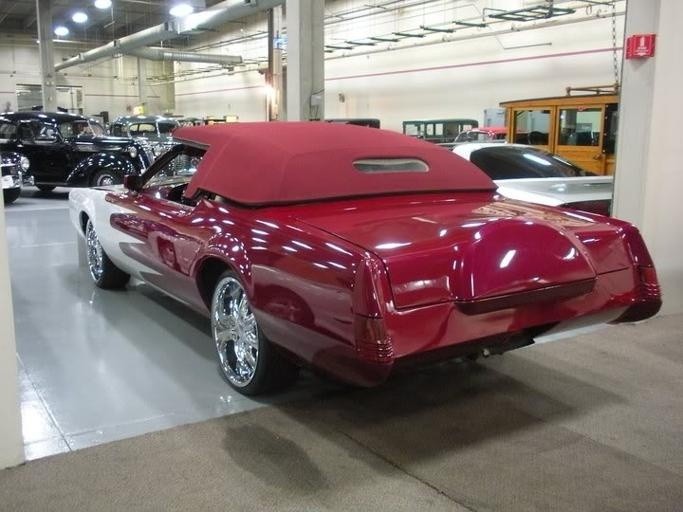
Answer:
[0,111,196,205]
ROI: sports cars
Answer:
[433,142,614,214]
[67,122,664,396]
